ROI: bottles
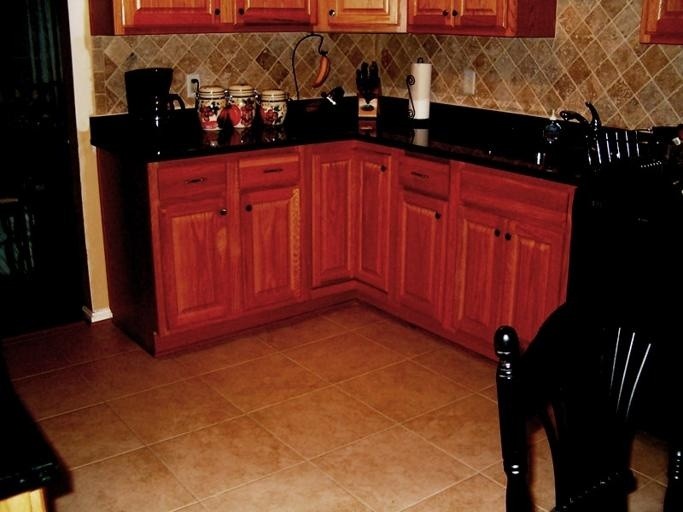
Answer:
[196,85,226,132]
[260,89,288,126]
[226,84,258,129]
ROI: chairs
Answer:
[492,289,682,511]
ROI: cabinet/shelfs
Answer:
[317,0,406,31]
[313,139,355,287]
[392,148,450,337]
[447,158,570,351]
[642,1,683,45]
[355,141,391,296]
[158,139,309,332]
[118,0,314,31]
[408,0,557,40]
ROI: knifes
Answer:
[355,60,379,88]
